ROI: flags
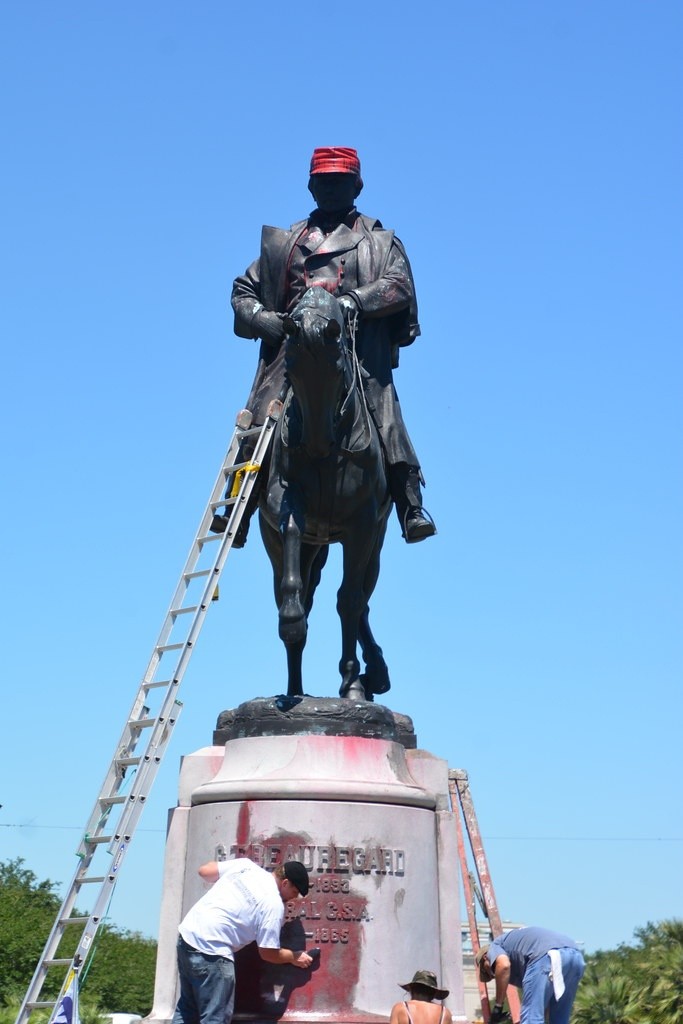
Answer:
[52,969,80,1024]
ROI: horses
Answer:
[255,286,395,702]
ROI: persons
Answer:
[175,861,313,1024]
[389,971,452,1024]
[229,146,436,544]
[475,926,585,1024]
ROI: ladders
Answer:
[446,766,525,1024]
[13,398,284,1024]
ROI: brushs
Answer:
[307,947,320,959]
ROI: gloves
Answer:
[487,1005,503,1024]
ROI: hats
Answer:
[475,943,492,983]
[284,859,310,897]
[396,970,450,1000]
